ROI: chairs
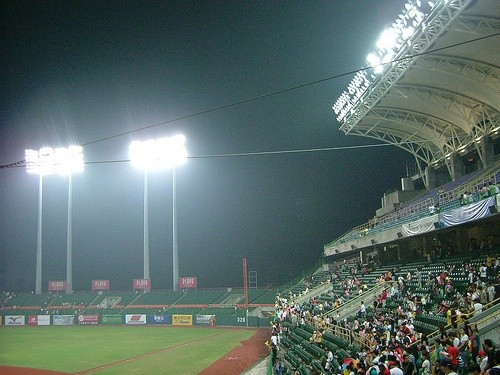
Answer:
[0,287,276,319]
[276,250,500,375]
[328,161,500,246]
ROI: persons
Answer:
[445,188,449,196]
[457,183,498,204]
[266,234,500,375]
[437,187,443,198]
[435,203,441,213]
[368,218,373,228]
[0,291,144,316]
[372,216,379,226]
[399,200,403,210]
[427,202,435,215]
